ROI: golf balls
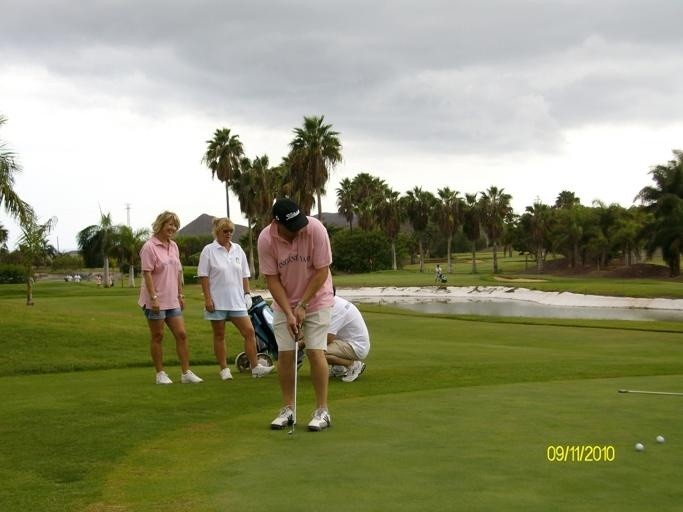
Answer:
[636,443,644,452]
[656,436,664,443]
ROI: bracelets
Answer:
[245,292,250,293]
[151,296,158,300]
[179,295,184,298]
[298,301,306,309]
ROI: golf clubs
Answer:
[288,325,299,434]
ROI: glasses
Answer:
[222,229,233,233]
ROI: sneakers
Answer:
[219,367,233,381]
[251,364,275,378]
[328,366,347,377]
[341,361,367,382]
[271,405,296,430]
[181,370,204,383]
[155,371,173,385]
[307,409,331,431]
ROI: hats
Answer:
[272,198,309,232]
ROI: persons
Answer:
[323,286,370,382]
[435,265,447,283]
[197,217,275,380]
[137,211,203,385]
[257,199,336,431]
[66,272,113,288]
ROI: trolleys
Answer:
[437,273,447,283]
[234,294,303,375]
